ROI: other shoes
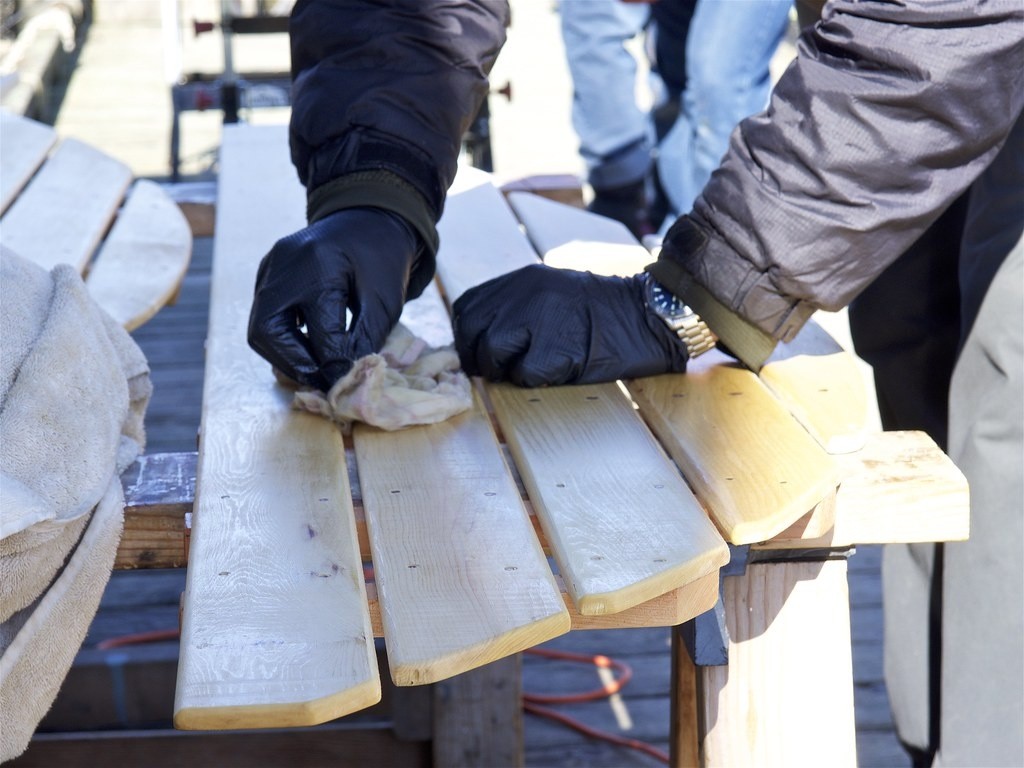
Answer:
[582,168,669,239]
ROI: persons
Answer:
[245,0,1023,764]
[553,0,790,233]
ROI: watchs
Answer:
[644,271,715,359]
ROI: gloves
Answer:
[452,265,689,387]
[247,207,424,394]
